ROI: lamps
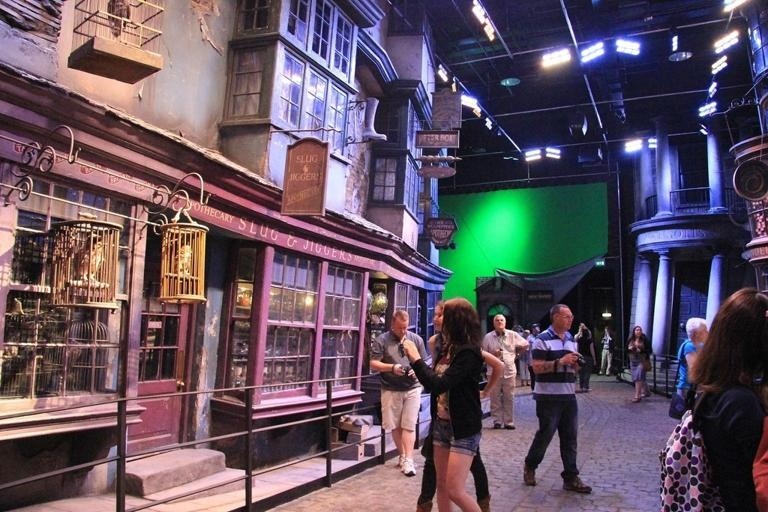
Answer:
[499,59,520,87]
[667,28,695,63]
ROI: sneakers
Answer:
[505,424,516,430]
[493,422,501,429]
[597,372,610,377]
[580,385,593,394]
[524,461,537,486]
[402,458,417,477]
[398,454,405,467]
[563,477,592,493]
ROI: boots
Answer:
[476,494,491,512]
[416,496,433,512]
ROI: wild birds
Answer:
[75,241,108,282]
[13,298,24,315]
[175,245,192,276]
[108,0,130,37]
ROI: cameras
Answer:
[401,366,410,376]
[577,355,587,367]
[399,343,404,358]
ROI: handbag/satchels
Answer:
[669,394,688,419]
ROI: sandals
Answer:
[632,392,652,403]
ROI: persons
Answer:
[625,326,653,403]
[658,287,768,512]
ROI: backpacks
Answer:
[659,392,725,512]
